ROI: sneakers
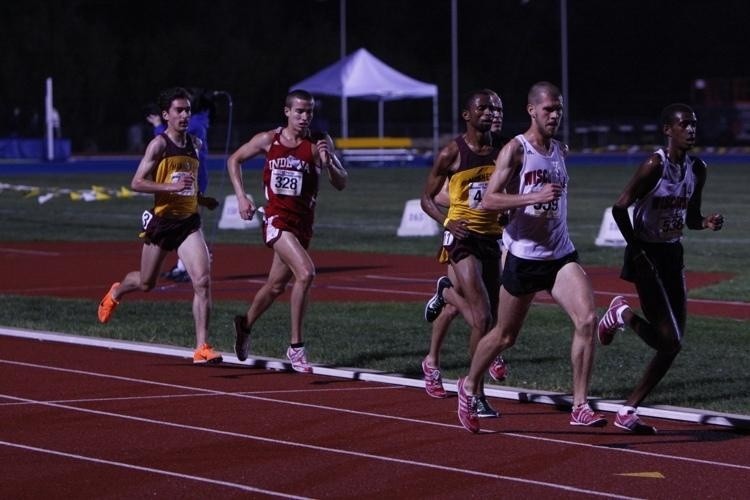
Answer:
[287,345,313,374]
[614,412,658,435]
[570,402,608,427]
[98,282,122,324]
[234,315,250,361]
[457,376,502,433]
[425,276,453,322]
[422,355,448,399]
[193,344,222,365]
[489,354,508,382]
[598,297,630,345]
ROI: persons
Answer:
[597,103,724,435]
[147,88,209,282]
[232,88,348,375]
[458,80,608,434]
[97,88,223,366]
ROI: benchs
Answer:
[342,149,416,167]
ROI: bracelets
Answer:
[443,217,450,227]
[633,250,646,262]
